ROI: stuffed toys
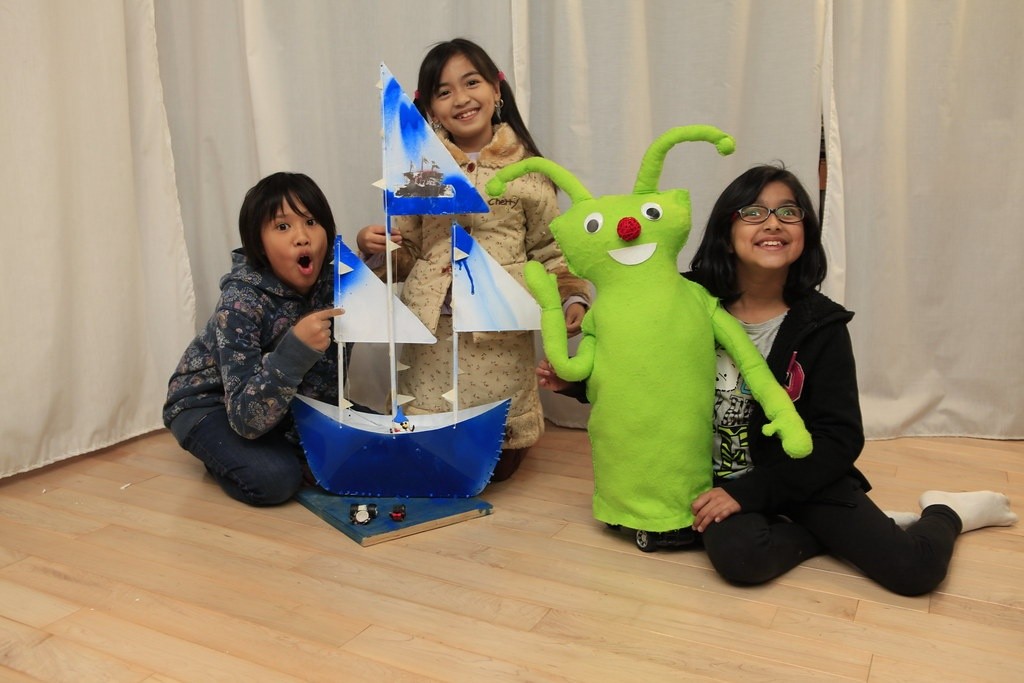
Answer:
[486,123,813,533]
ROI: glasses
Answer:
[730,205,806,225]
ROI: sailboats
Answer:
[290,61,544,500]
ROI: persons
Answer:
[161,170,361,506]
[342,37,593,484]
[534,158,1019,597]
